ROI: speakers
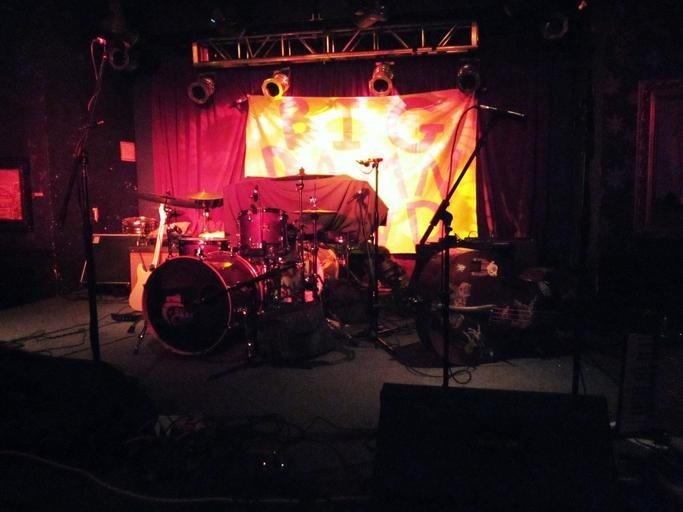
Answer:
[380,382,617,484]
[1,347,153,471]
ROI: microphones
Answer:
[96,37,131,70]
[481,104,529,123]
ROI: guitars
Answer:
[129,202,170,312]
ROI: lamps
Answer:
[180,60,397,105]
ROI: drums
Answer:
[262,241,339,307]
[240,208,287,256]
[179,237,232,256]
[121,216,158,246]
[142,251,264,356]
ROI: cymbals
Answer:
[291,209,337,213]
[271,176,334,179]
[187,193,223,200]
[125,193,200,208]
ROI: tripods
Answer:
[248,165,416,353]
